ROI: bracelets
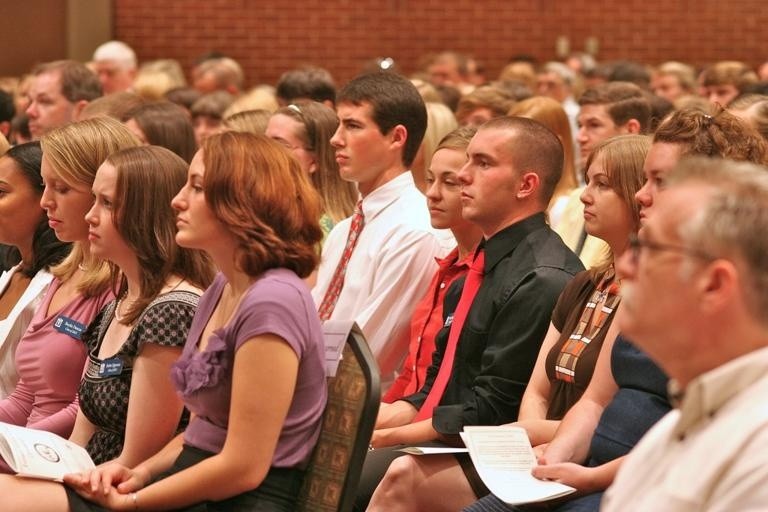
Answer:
[130,494,140,510]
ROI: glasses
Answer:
[624,232,717,266]
[279,140,316,157]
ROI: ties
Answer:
[410,247,487,430]
[316,199,365,324]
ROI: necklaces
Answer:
[77,262,90,272]
[206,282,250,329]
[115,276,187,323]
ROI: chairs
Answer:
[294,318,382,512]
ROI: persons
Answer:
[457,85,505,121]
[725,96,768,138]
[264,97,360,294]
[361,134,652,511]
[360,116,587,512]
[363,123,484,399]
[277,68,337,112]
[650,100,674,126]
[190,96,229,142]
[462,100,768,512]
[77,87,138,124]
[600,156,768,510]
[196,55,243,92]
[122,102,195,162]
[24,59,101,141]
[162,86,196,125]
[218,109,270,136]
[505,97,575,208]
[547,80,650,273]
[2,131,327,510]
[93,40,134,93]
[2,116,141,472]
[0,147,214,479]
[139,60,185,101]
[312,70,443,388]
[0,144,55,410]
[412,103,458,190]
